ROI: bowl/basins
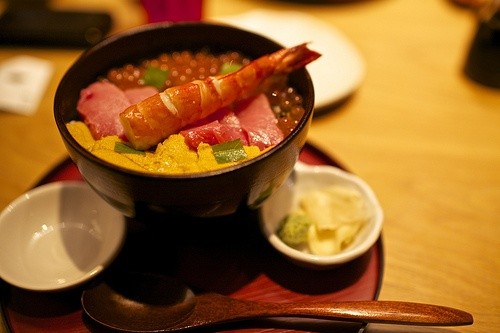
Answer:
[52,20,315,230]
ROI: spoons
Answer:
[81,267,474,333]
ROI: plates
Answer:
[212,10,364,112]
[259,159,384,264]
[0,179,126,291]
